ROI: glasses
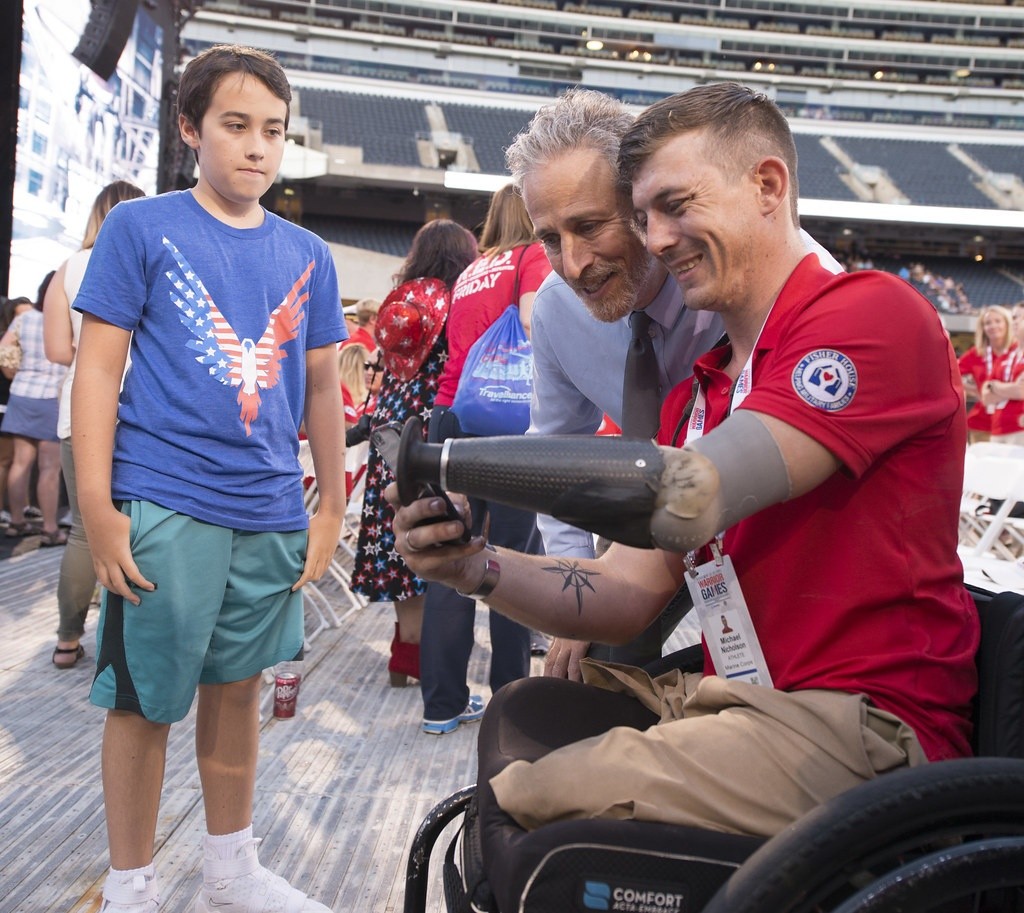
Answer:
[363,360,384,374]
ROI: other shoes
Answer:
[529,629,552,655]
[23,505,44,517]
[0,510,12,522]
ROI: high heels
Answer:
[389,623,420,688]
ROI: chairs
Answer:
[259,434,371,720]
[959,438,1024,589]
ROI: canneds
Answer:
[273,672,298,720]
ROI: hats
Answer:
[374,278,450,382]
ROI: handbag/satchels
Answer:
[343,414,374,448]
[0,317,21,379]
[449,244,534,436]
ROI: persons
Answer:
[421,181,541,734]
[503,85,847,687]
[378,86,980,912]
[346,218,479,685]
[72,45,347,913]
[1,181,148,670]
[841,249,1024,448]
[298,294,385,508]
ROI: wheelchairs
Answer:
[404,582,1024,913]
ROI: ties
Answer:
[622,312,662,441]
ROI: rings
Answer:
[405,529,420,552]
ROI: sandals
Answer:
[52,644,84,668]
[5,523,41,537]
[40,528,68,546]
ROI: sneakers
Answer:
[100,875,160,913]
[200,841,333,913]
[420,695,486,735]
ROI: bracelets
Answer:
[456,542,500,601]
